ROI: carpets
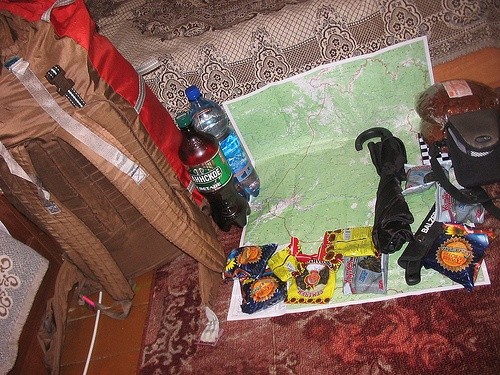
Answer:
[138,178,499,375]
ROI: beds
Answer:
[82,0,500,125]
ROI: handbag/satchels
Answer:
[444,109,500,189]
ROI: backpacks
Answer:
[1,9,228,319]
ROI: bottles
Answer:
[175,113,251,231]
[185,85,260,202]
